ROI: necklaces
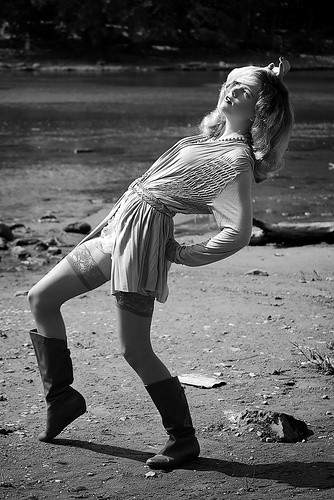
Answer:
[213,137,252,142]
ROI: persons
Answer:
[26,57,293,471]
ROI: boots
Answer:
[29,328,87,441]
[143,376,200,468]
